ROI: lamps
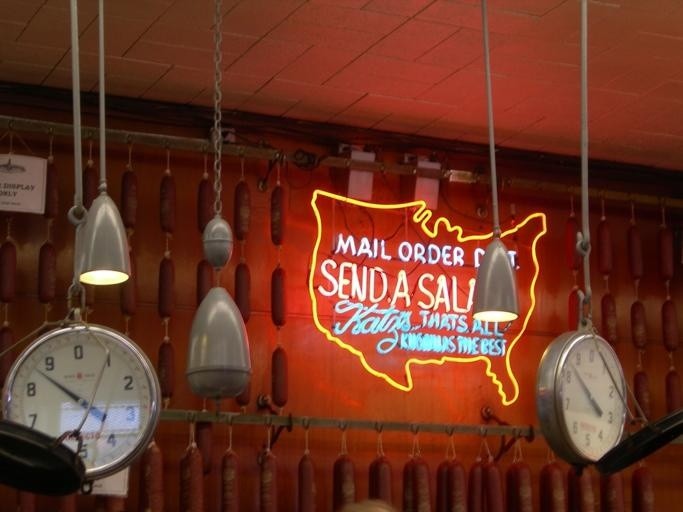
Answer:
[185,0,252,399]
[475,0,518,323]
[79,1,131,287]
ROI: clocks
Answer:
[536,290,630,468]
[3,309,162,486]
